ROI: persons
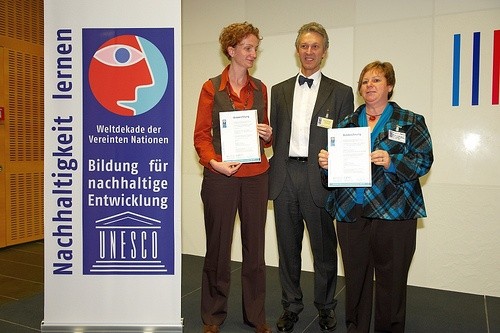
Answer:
[318,61,434,333]
[268,22,354,333]
[193,22,273,333]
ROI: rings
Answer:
[382,158,384,161]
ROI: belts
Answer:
[288,156,308,163]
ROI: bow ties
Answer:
[298,75,313,88]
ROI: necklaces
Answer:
[366,113,381,121]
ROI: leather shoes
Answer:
[318,308,337,332]
[276,312,299,333]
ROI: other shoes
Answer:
[256,323,272,333]
[203,324,220,333]
